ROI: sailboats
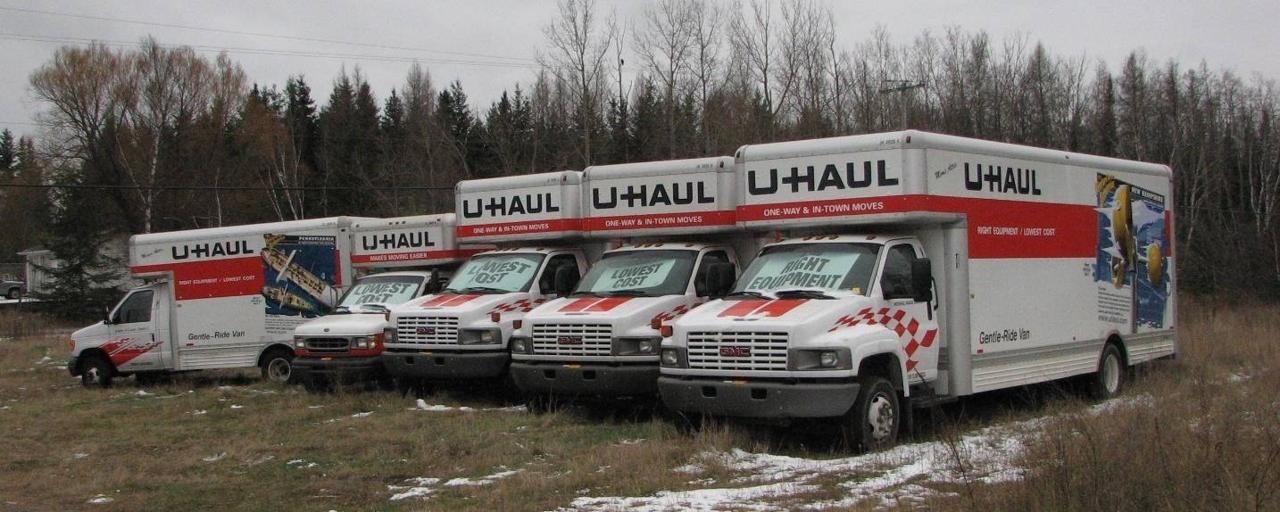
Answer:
[260,247,341,308]
[262,286,315,313]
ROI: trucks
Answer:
[651,126,1183,458]
[292,211,463,403]
[66,211,375,394]
[379,165,607,410]
[509,151,735,420]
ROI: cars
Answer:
[0,278,25,300]
[0,273,19,280]
